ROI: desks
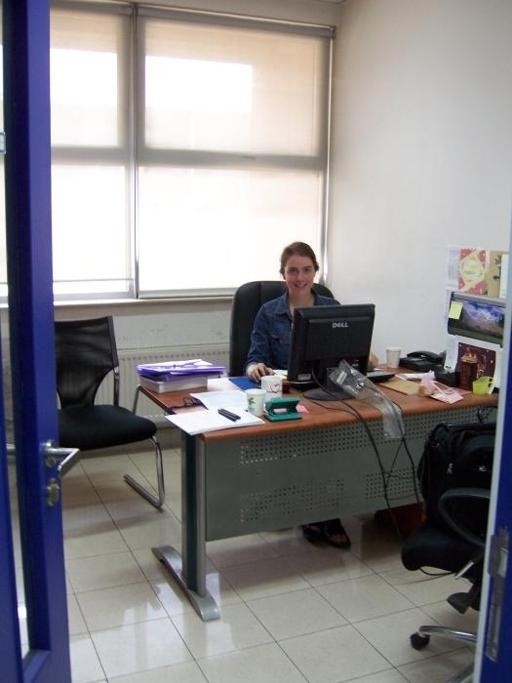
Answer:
[138,363,500,622]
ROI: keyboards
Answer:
[283,368,394,377]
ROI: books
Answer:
[138,376,209,395]
[165,408,262,436]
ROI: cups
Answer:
[262,375,282,402]
[246,388,266,418]
[386,347,401,369]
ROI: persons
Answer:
[245,242,379,549]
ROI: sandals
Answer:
[304,522,330,548]
[325,519,352,550]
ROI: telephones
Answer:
[399,351,444,373]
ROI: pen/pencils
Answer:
[217,408,240,421]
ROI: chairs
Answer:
[228,281,335,377]
[401,422,497,650]
[53,313,166,509]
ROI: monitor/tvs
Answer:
[287,304,376,401]
